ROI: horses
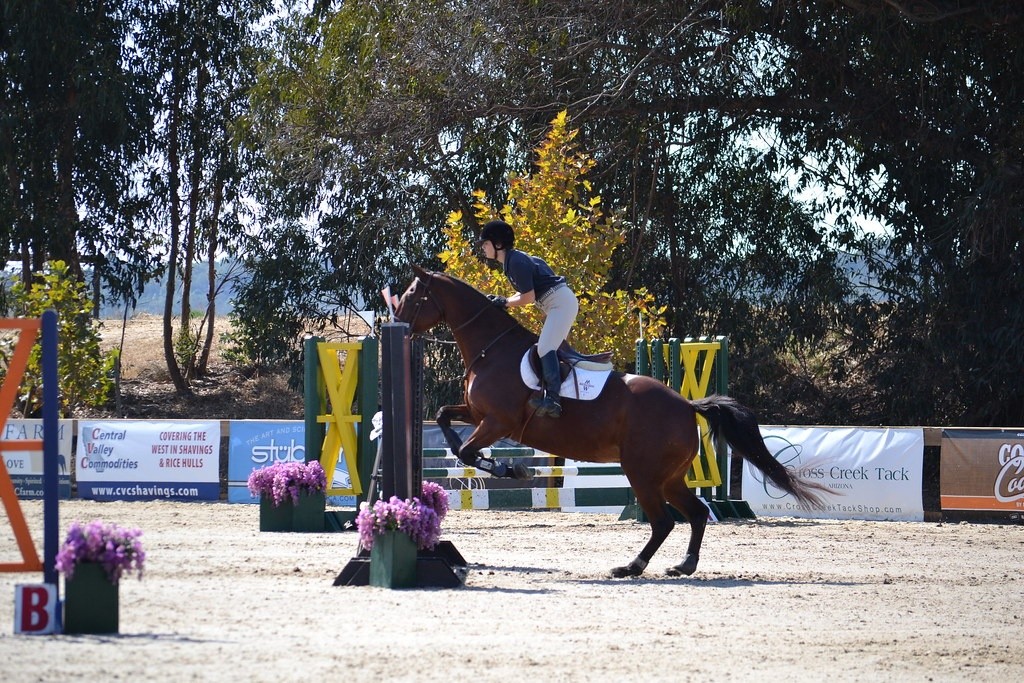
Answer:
[386,263,847,582]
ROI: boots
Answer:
[529,351,565,419]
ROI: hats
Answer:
[474,221,515,249]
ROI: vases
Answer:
[369,529,418,588]
[64,561,119,634]
[258,486,326,534]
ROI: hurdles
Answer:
[333,322,757,588]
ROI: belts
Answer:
[540,283,568,302]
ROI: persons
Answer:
[479,221,579,418]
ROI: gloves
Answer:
[487,294,507,310]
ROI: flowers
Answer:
[420,480,449,519]
[54,519,146,581]
[246,459,326,508]
[355,495,441,551]
